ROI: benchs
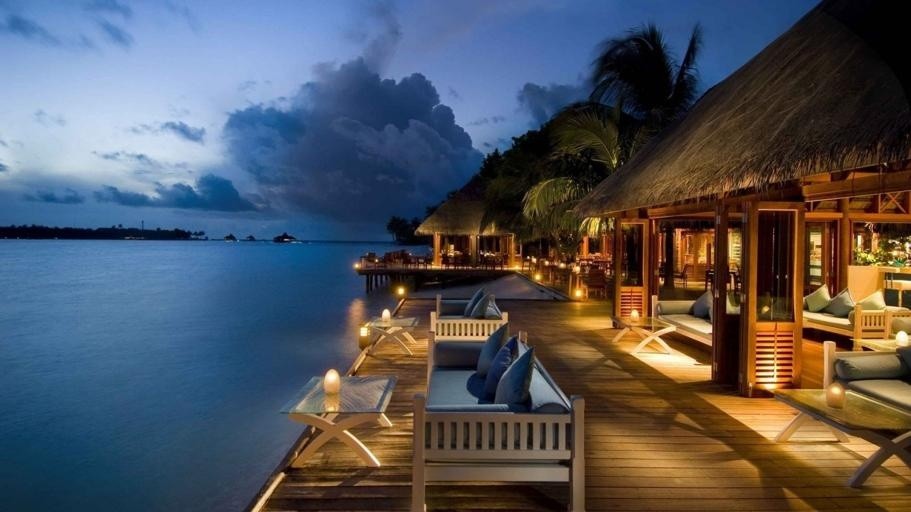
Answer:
[409,326,588,510]
[821,337,910,413]
[647,289,744,349]
[796,287,895,342]
[428,287,509,342]
[359,243,743,300]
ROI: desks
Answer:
[613,315,675,359]
[362,315,420,358]
[276,373,399,472]
[767,386,909,493]
[852,338,908,355]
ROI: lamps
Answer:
[381,308,391,322]
[823,382,846,405]
[322,368,341,395]
[629,309,640,321]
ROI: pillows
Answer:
[710,294,733,319]
[493,344,536,405]
[475,322,510,375]
[825,290,853,319]
[464,287,485,316]
[809,283,830,311]
[470,293,491,317]
[688,289,715,318]
[482,334,519,397]
[849,287,887,314]
